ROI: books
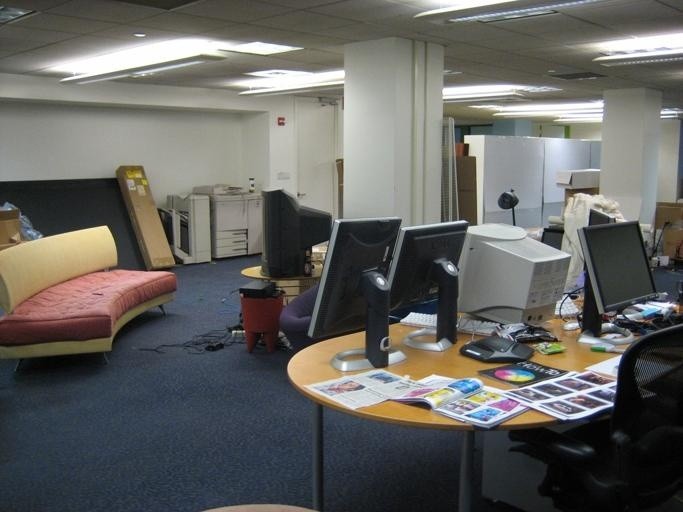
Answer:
[584,352,674,388]
[388,371,532,429]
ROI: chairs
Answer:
[505,320,683,511]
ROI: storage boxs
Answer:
[449,155,477,230]
[0,207,24,245]
[117,165,176,271]
[655,203,683,260]
[553,168,601,210]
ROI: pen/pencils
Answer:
[590,346,625,354]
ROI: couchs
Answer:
[0,224,178,372]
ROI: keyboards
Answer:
[399,310,500,338]
[554,291,579,319]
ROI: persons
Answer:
[320,381,365,396]
[516,376,613,414]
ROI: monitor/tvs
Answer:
[580,207,617,272]
[386,218,470,351]
[458,221,572,328]
[304,216,403,373]
[258,186,333,280]
[579,218,659,338]
[542,228,565,249]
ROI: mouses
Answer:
[562,320,580,330]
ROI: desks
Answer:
[288,292,683,510]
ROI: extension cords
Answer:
[231,330,247,338]
